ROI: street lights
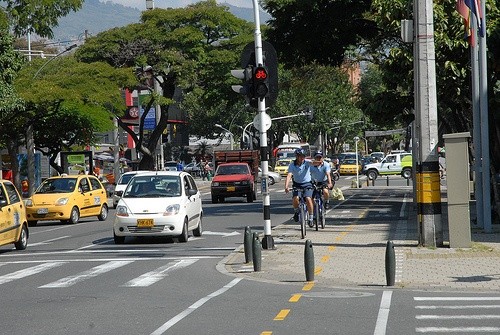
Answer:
[27,43,77,199]
[240,112,314,148]
[215,124,234,150]
[324,120,365,157]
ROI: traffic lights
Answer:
[254,65,269,99]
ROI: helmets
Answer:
[314,149,323,157]
[295,148,306,155]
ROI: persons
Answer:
[334,156,340,174]
[75,161,212,192]
[285,148,323,228]
[308,153,333,223]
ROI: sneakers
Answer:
[294,209,300,222]
[325,203,329,209]
[309,218,313,227]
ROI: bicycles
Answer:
[287,184,310,238]
[310,179,328,231]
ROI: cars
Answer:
[77,156,212,185]
[258,140,413,186]
[0,179,31,250]
[113,171,142,205]
[112,170,203,244]
[25,174,109,226]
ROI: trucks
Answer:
[208,149,258,204]
